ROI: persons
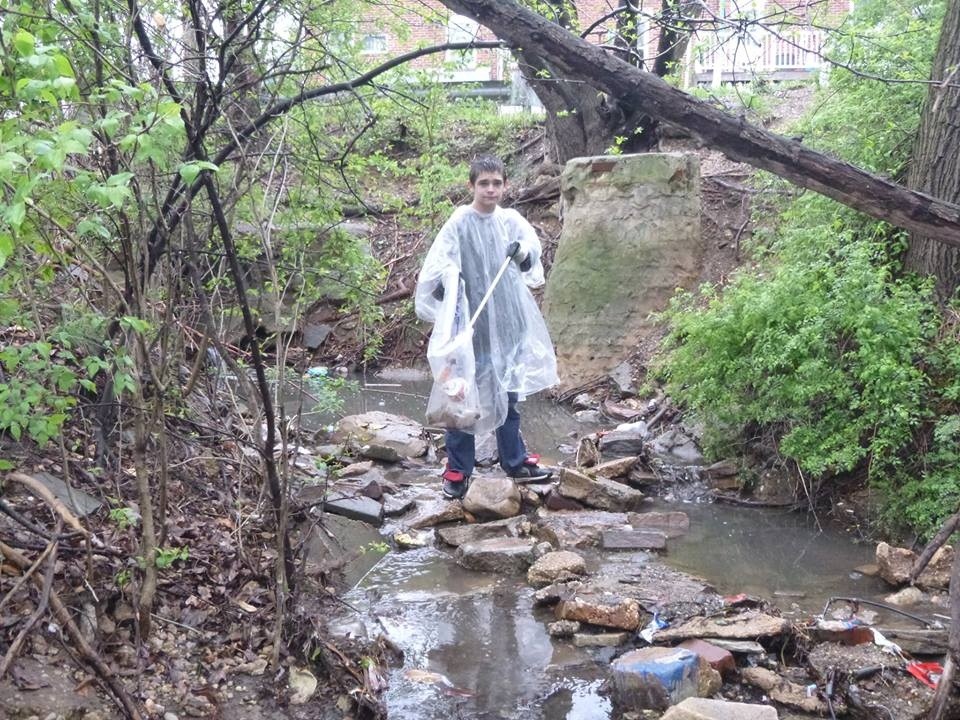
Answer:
[414,157,561,499]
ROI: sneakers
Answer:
[507,455,553,483]
[441,469,467,498]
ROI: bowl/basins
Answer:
[442,377,469,402]
